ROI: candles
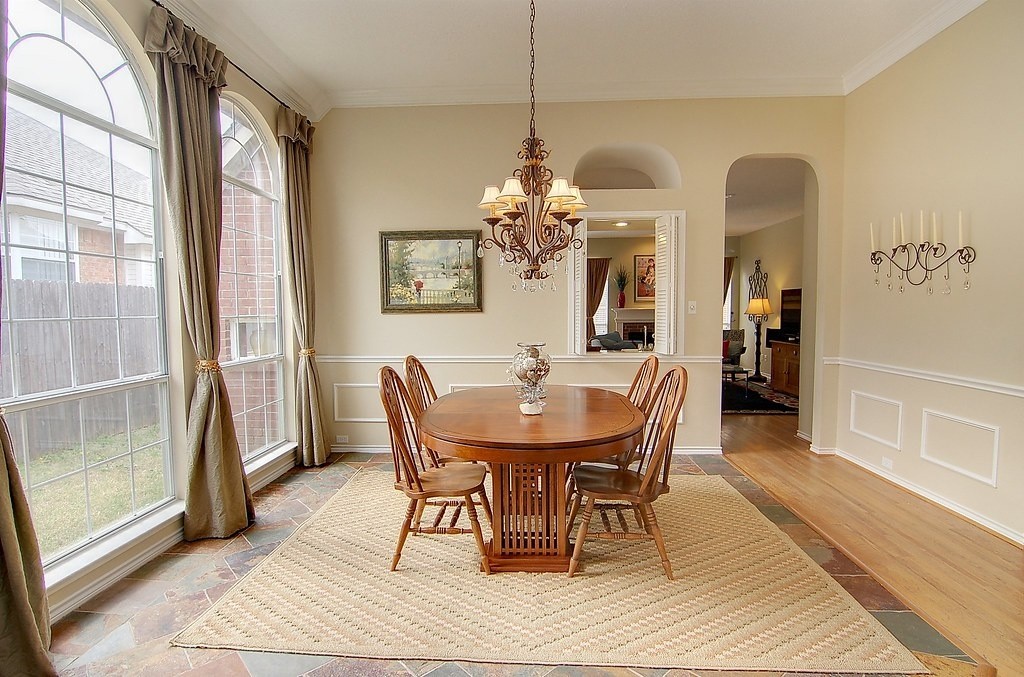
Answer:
[958,209,964,250]
[644,325,647,347]
[933,211,938,247]
[870,223,875,252]
[893,216,896,247]
[920,210,924,244]
[900,213,905,244]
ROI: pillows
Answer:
[722,339,743,360]
[590,331,636,350]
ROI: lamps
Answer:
[611,221,631,227]
[476,1,591,294]
[744,298,774,383]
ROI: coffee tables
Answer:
[721,368,752,399]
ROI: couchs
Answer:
[586,331,643,353]
[721,329,747,366]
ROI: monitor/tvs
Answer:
[781,288,801,336]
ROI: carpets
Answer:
[168,471,931,674]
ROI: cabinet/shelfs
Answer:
[769,340,802,397]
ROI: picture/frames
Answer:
[377,229,484,315]
[633,254,656,303]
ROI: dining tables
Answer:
[416,385,645,572]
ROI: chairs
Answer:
[565,365,688,580]
[565,355,659,529]
[401,355,491,524]
[377,366,495,576]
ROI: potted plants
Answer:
[610,262,632,309]
